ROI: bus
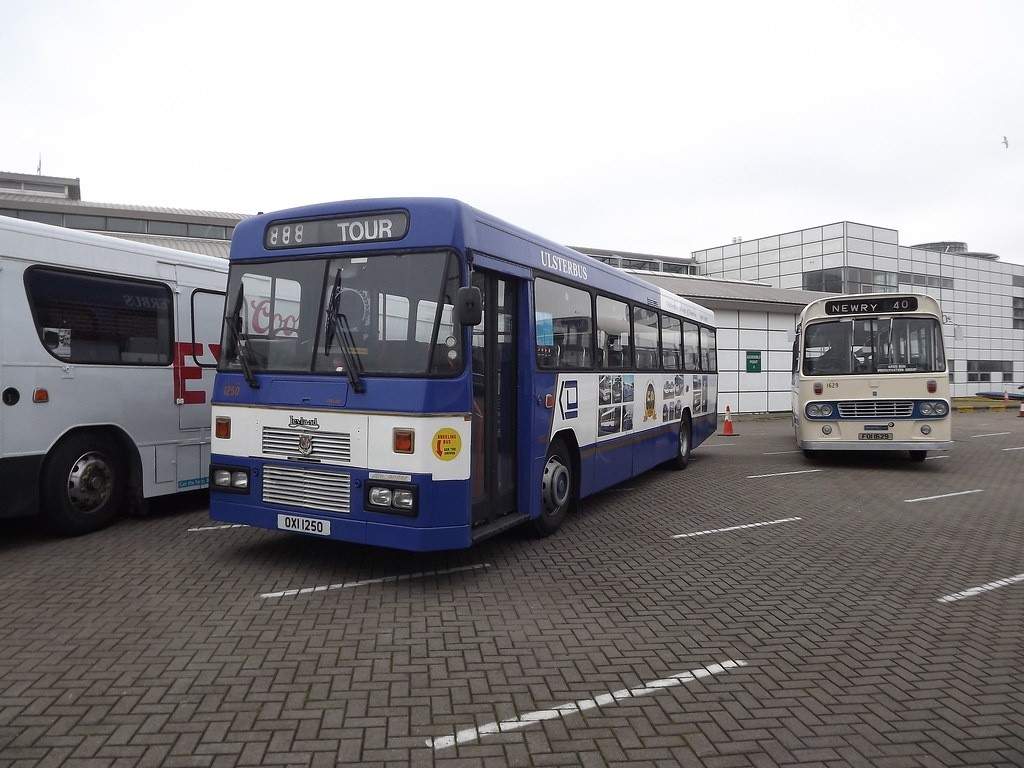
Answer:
[789,292,962,462]
[204,199,720,556]
[0,214,505,535]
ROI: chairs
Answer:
[537,344,709,371]
[125,337,161,364]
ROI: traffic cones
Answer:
[1017,399,1024,417]
[718,406,740,437]
[1003,390,1009,403]
